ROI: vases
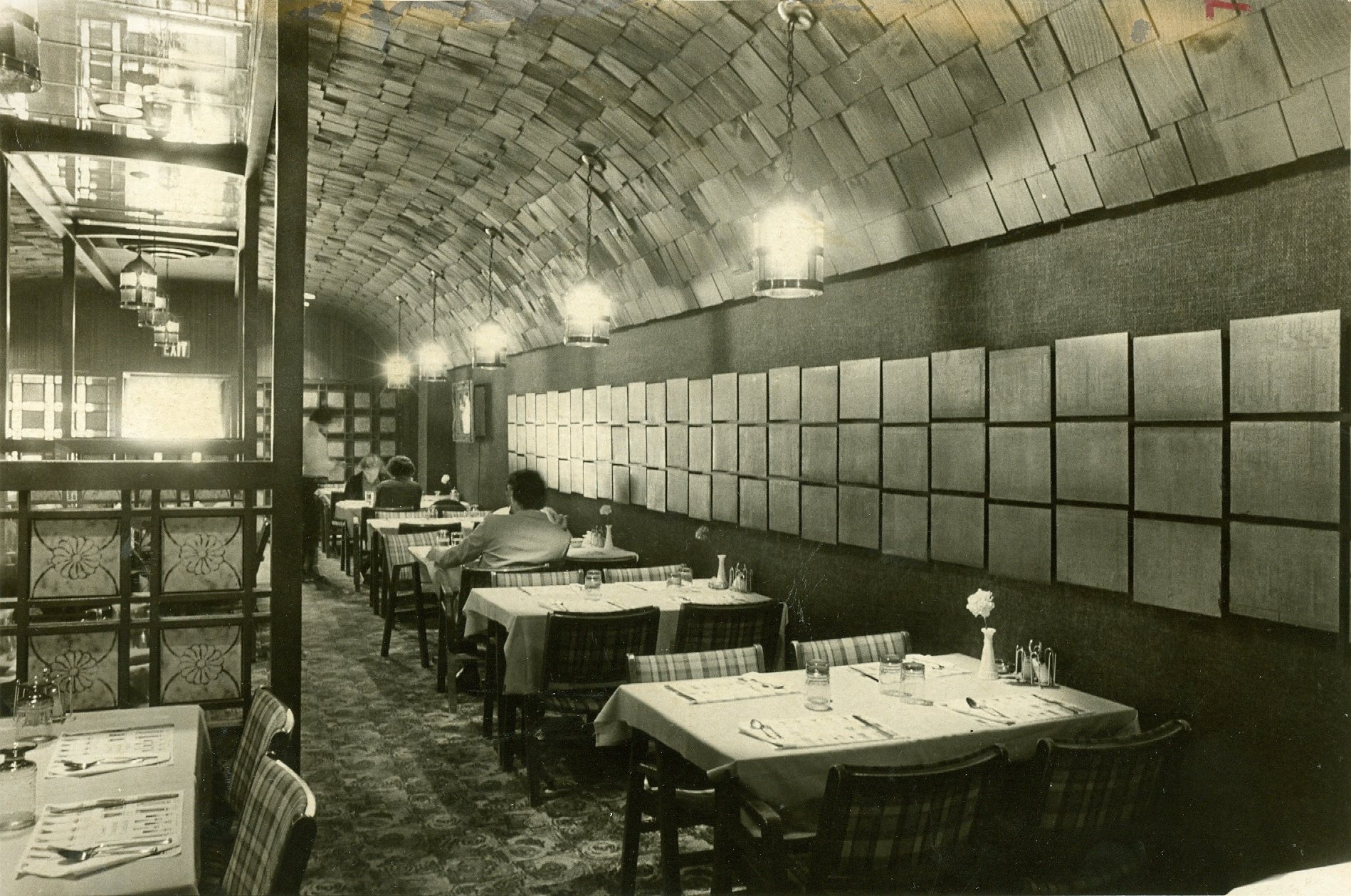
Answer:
[717,554,726,586]
[603,525,614,553]
[450,490,456,501]
[978,627,999,680]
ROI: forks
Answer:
[60,756,159,765]
[47,838,172,853]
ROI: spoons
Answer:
[752,719,776,740]
[966,697,999,719]
[65,761,144,769]
[58,847,158,861]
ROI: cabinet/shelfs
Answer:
[256,383,400,534]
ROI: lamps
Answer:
[472,227,506,370]
[751,0,825,298]
[120,209,179,347]
[388,296,410,389]
[419,270,447,382]
[563,155,610,348]
[0,0,41,98]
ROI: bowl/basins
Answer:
[571,538,582,548]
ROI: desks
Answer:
[407,537,640,671]
[335,495,458,576]
[462,578,788,766]
[0,704,212,896]
[366,517,490,614]
[592,652,1141,896]
[314,488,345,558]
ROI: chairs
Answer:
[200,493,1194,896]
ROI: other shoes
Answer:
[456,667,479,691]
[311,572,327,581]
[301,574,316,583]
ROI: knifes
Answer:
[1033,694,1079,714]
[665,685,697,701]
[851,667,880,682]
[853,715,894,738]
[50,794,180,814]
[65,722,174,736]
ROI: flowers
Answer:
[695,526,721,555]
[599,504,612,525]
[441,473,455,491]
[966,588,995,629]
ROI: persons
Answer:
[426,470,572,688]
[302,408,337,582]
[460,392,471,434]
[345,455,422,589]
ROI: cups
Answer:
[451,533,462,547]
[365,491,372,506]
[438,530,448,549]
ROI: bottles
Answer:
[902,664,926,704]
[587,570,602,577]
[680,568,693,585]
[667,572,681,587]
[585,575,601,601]
[0,742,37,831]
[879,652,901,696]
[805,660,830,711]
[14,667,73,745]
